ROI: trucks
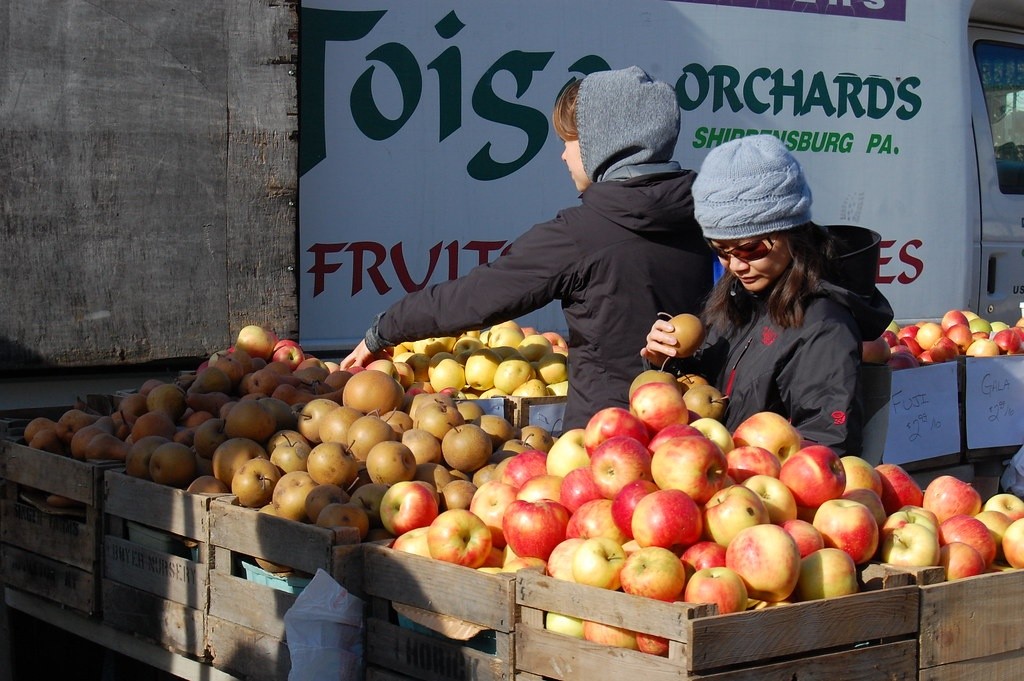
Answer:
[1,0,1023,490]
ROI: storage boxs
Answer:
[0,353,1024,681]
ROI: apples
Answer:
[194,319,569,399]
[380,381,1024,656]
[860,308,1024,370]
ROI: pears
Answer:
[628,312,729,421]
[23,353,556,572]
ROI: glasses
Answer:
[708,233,782,263]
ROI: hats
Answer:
[691,135,813,238]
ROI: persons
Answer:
[342,67,713,438]
[644,133,894,468]
